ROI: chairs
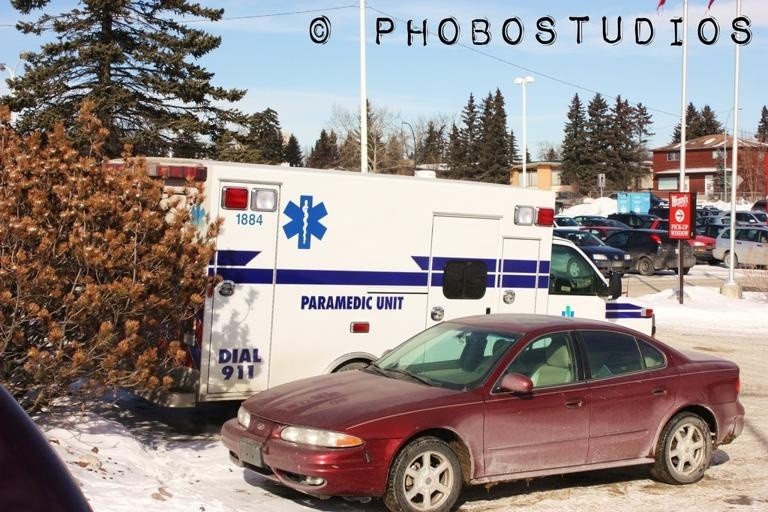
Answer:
[524,340,572,388]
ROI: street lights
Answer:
[0,51,28,127]
[400,121,418,172]
[513,74,536,188]
[722,107,744,202]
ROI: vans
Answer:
[711,225,767,269]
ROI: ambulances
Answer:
[88,153,657,429]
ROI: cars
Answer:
[553,224,632,280]
[555,192,767,237]
[216,311,744,512]
[603,228,697,277]
[641,219,721,267]
